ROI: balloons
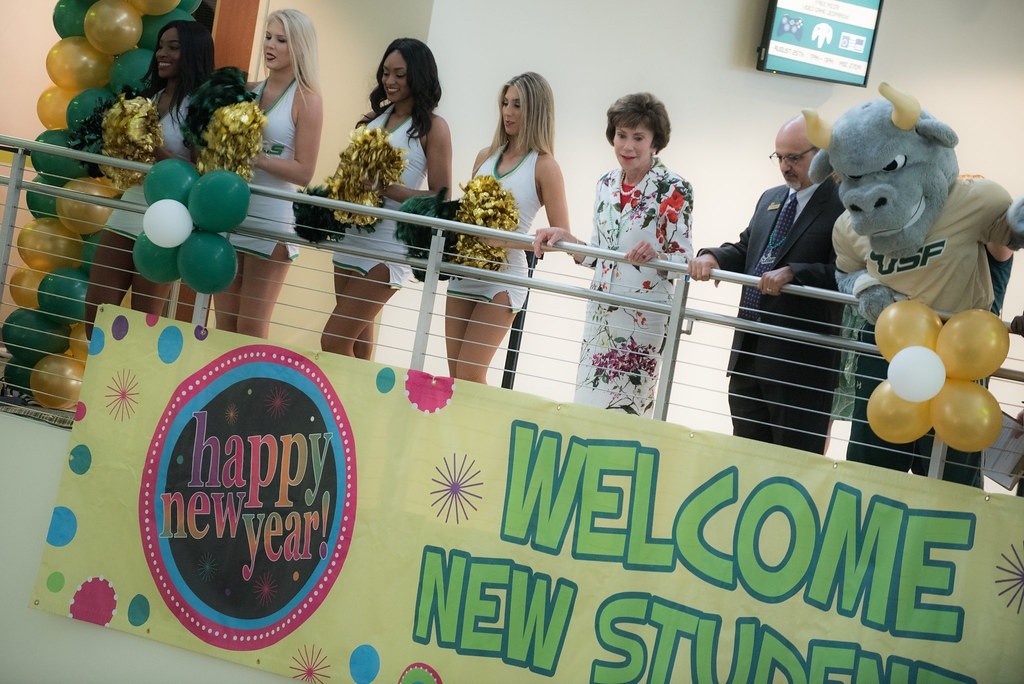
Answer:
[866,300,1010,453]
[0,0,250,413]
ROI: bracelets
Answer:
[568,238,579,256]
[656,251,660,261]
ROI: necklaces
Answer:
[620,157,655,196]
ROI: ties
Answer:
[740,191,798,322]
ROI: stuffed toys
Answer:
[801,82,1024,488]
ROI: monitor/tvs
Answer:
[756,0,884,89]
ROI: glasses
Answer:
[769,147,817,167]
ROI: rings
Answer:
[641,254,647,259]
[766,287,772,292]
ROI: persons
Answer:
[445,72,567,384]
[533,91,694,415]
[321,36,452,360]
[83,19,215,346]
[212,9,324,340]
[686,115,848,455]
